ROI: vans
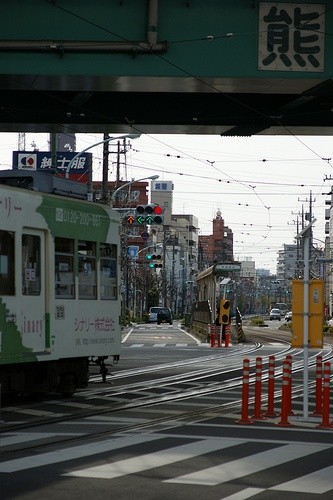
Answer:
[270,308,281,321]
[156,307,173,325]
[149,306,162,322]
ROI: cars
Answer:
[284,311,292,320]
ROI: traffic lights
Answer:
[144,253,163,261]
[137,203,165,216]
[219,300,232,324]
[150,263,163,268]
[124,213,165,227]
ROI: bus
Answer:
[275,302,289,317]
[0,169,123,401]
